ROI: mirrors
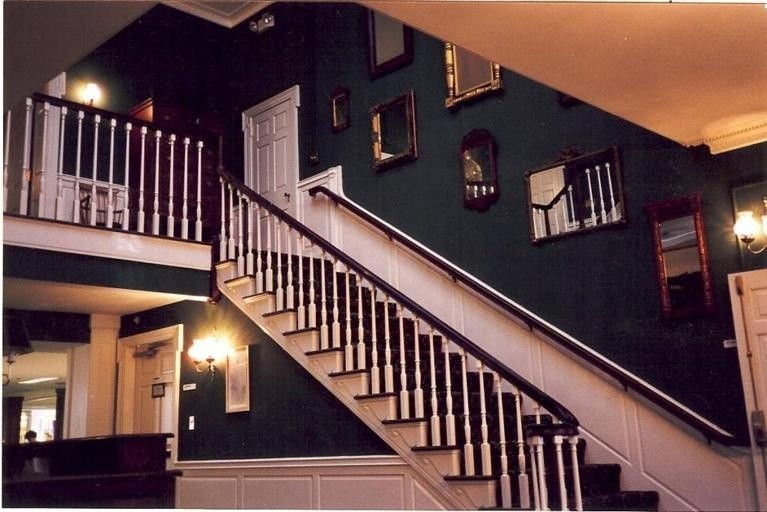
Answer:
[368,4,413,82]
[461,125,498,213]
[525,143,624,245]
[369,87,418,174]
[443,44,501,106]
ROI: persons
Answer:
[24,430,36,442]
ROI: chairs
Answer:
[77,181,124,231]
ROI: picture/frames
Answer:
[332,89,350,130]
[224,345,252,413]
[642,196,714,314]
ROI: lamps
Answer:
[733,193,767,254]
[3,316,36,386]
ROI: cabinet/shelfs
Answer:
[124,85,225,233]
